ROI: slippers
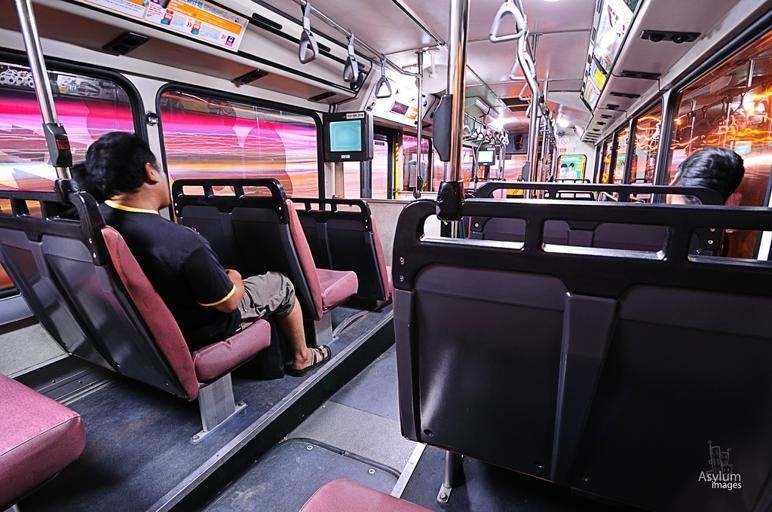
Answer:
[293,345,331,377]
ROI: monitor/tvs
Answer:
[323,110,373,161]
[477,149,496,166]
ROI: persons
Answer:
[650,142,748,257]
[559,162,569,181]
[83,128,330,375]
[568,162,577,178]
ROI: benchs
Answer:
[1,184,772,511]
